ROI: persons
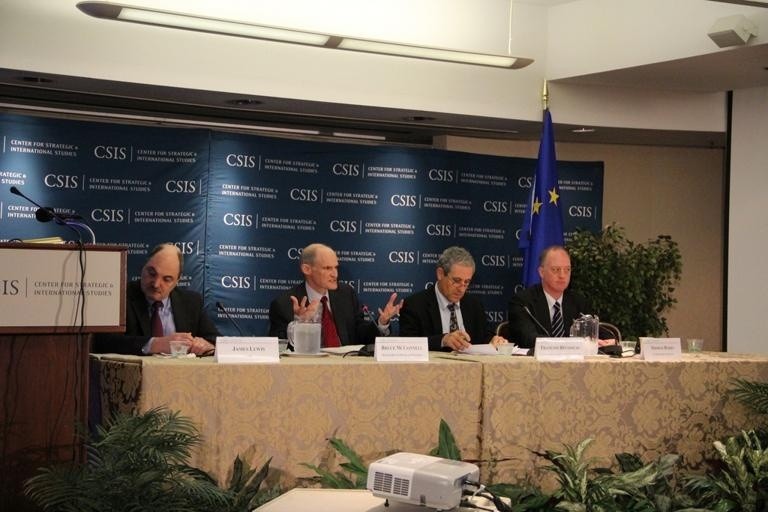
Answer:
[508,244,618,350]
[94,243,223,358]
[397,246,509,353]
[268,243,404,353]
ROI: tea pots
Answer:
[287,304,323,354]
[570,314,599,355]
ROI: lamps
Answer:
[77,0,535,70]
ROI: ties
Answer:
[446,302,459,333]
[150,299,164,337]
[550,301,567,337]
[318,295,340,348]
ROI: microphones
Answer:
[216,301,244,336]
[10,187,81,245]
[359,304,384,356]
[36,206,83,222]
[520,303,551,355]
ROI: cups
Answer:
[687,338,704,356]
[497,342,515,360]
[619,341,637,357]
[168,340,189,359]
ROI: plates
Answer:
[287,350,329,358]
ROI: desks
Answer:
[436,351,768,495]
[98,352,482,487]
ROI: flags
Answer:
[518,107,565,292]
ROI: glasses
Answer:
[444,269,471,288]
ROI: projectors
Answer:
[366,452,480,510]
